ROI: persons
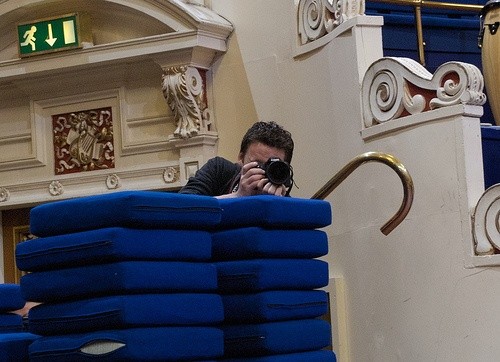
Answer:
[178,121,295,198]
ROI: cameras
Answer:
[254,157,293,189]
